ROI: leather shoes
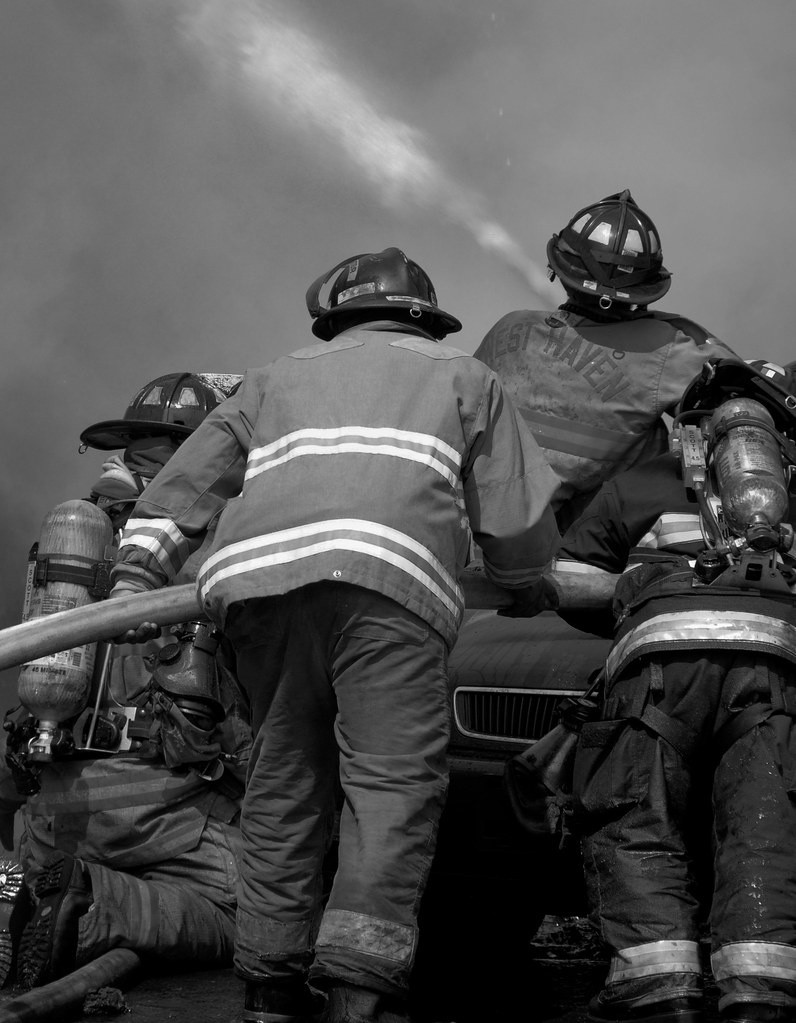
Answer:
[719,1002,796,1023]
[0,858,32,988]
[243,969,330,1023]
[587,995,700,1023]
[17,849,94,993]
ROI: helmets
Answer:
[306,247,462,342]
[546,188,671,309]
[78,372,227,454]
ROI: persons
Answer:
[2,189,795,1023]
